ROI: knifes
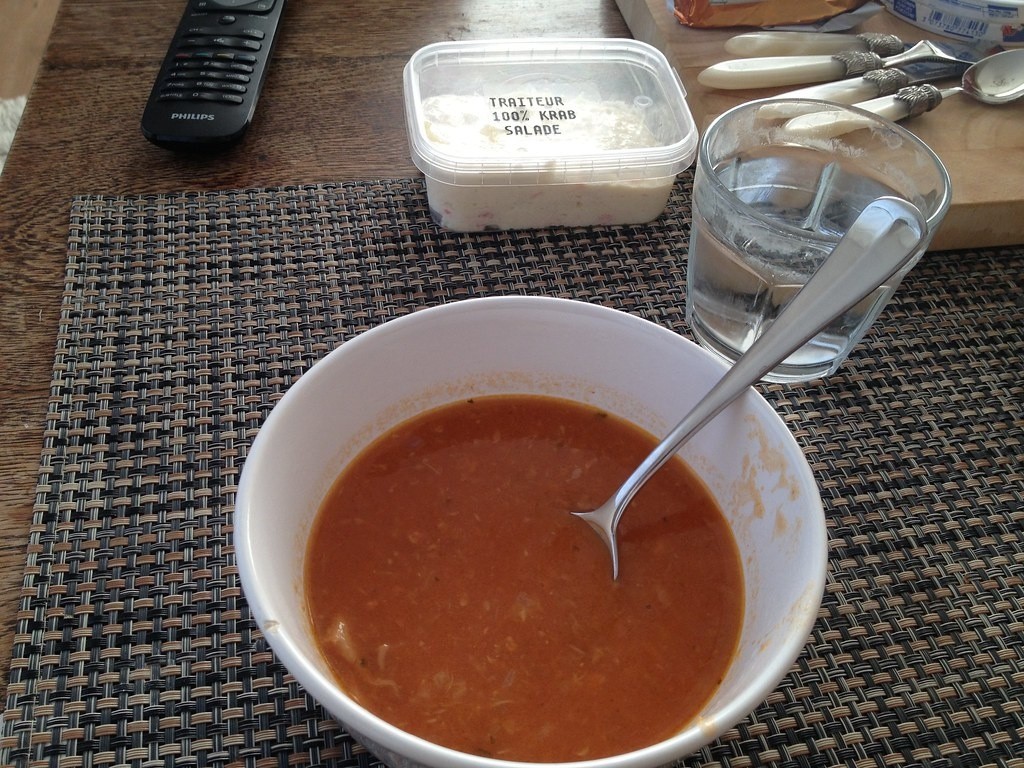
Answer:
[723,31,918,58]
[760,58,972,119]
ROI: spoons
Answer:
[782,48,1024,143]
[570,196,927,581]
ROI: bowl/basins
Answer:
[235,295,826,768]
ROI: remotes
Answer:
[142,0,288,150]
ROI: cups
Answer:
[686,97,952,383]
